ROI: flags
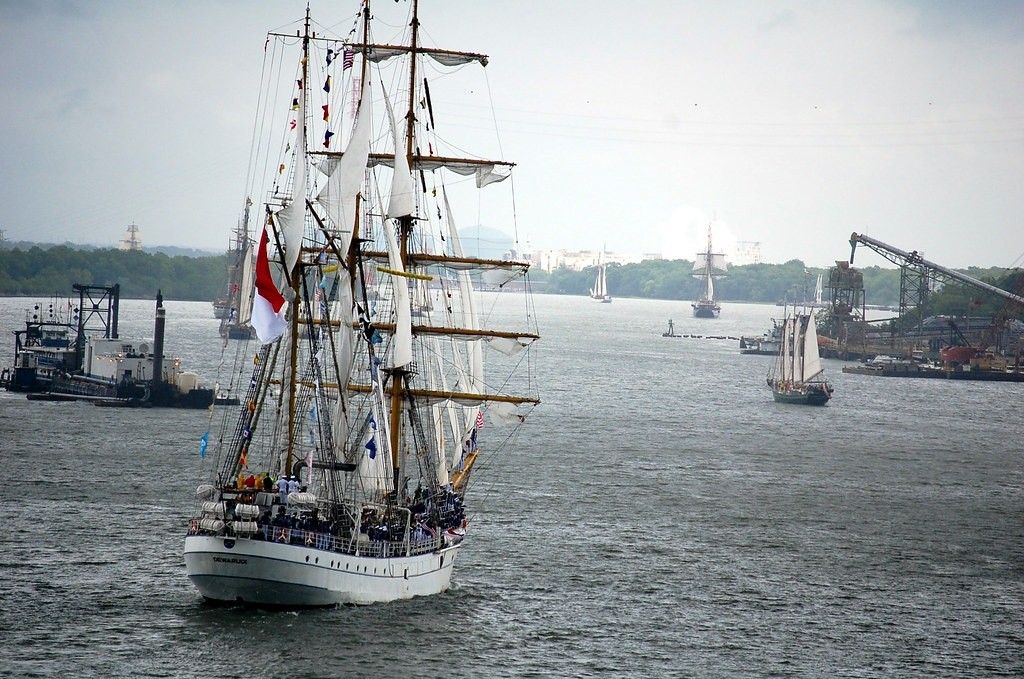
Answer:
[305,274,327,486]
[201,432,208,457]
[240,451,249,469]
[344,50,353,70]
[366,289,383,431]
[244,229,288,346]
[462,412,484,458]
[248,402,255,413]
[366,437,378,460]
[243,430,249,439]
[251,378,257,391]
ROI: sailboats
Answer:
[804,271,833,307]
[689,222,732,318]
[765,308,835,406]
[589,242,607,300]
[183,0,543,608]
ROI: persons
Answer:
[277,475,302,503]
[236,471,273,502]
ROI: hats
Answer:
[290,475,295,479]
[282,475,287,477]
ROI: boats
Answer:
[0,282,242,410]
[212,219,259,340]
[739,268,817,356]
